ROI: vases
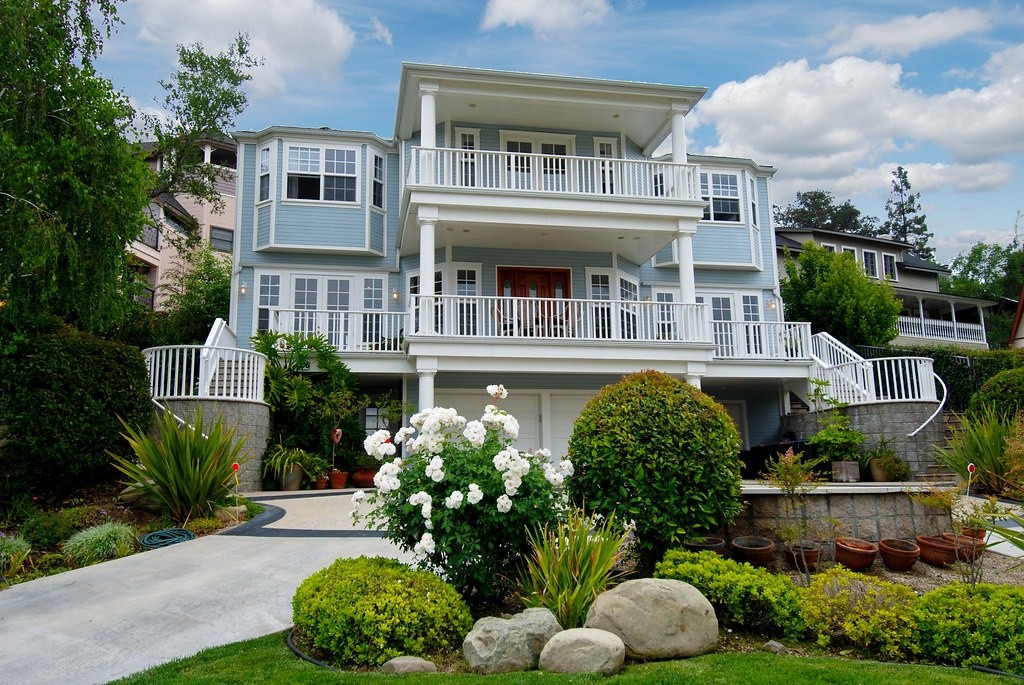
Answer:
[879,538,920,572]
[685,536,725,556]
[942,533,986,562]
[916,535,960,567]
[731,536,776,567]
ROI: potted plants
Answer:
[351,453,379,487]
[866,431,898,481]
[805,378,864,482]
[330,465,348,488]
[957,503,986,540]
[311,464,329,489]
[783,520,825,573]
[261,434,302,490]
[831,518,879,572]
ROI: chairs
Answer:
[548,302,580,336]
[517,300,545,336]
[491,307,521,336]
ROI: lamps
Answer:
[240,281,248,293]
[393,288,399,300]
[767,299,776,309]
